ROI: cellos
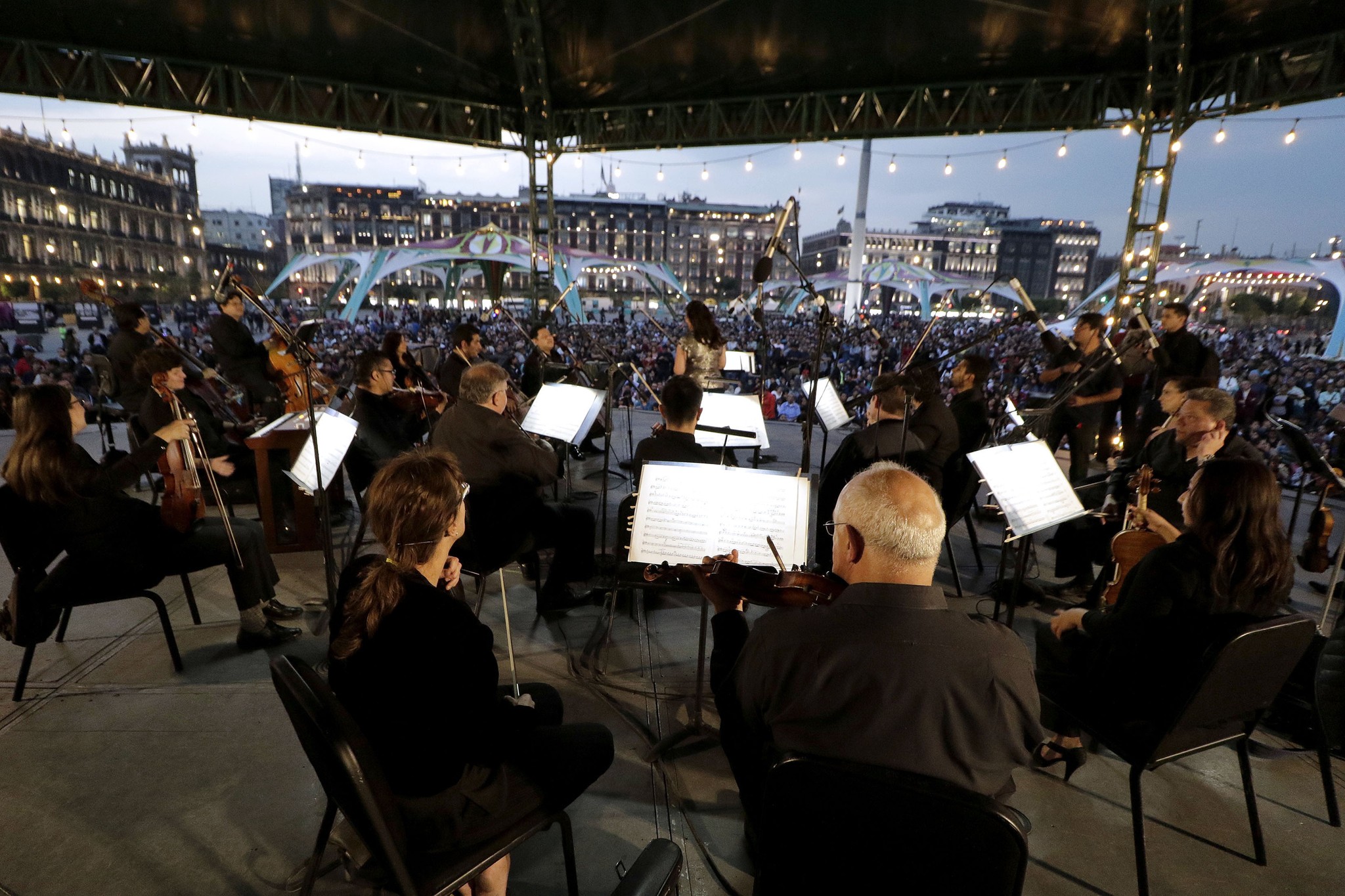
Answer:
[502,377,540,443]
[230,274,337,415]
[551,334,621,464]
[78,278,272,446]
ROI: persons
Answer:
[0,384,304,651]
[0,289,1345,617]
[327,448,613,896]
[1029,458,1296,782]
[684,460,1046,846]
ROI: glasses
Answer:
[823,519,848,535]
[377,370,396,375]
[444,481,471,538]
[68,398,86,408]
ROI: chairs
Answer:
[0,367,1345,896]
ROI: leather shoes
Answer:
[262,598,304,619]
[236,621,302,650]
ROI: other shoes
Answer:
[1042,579,1093,597]
[538,587,591,614]
[516,554,541,579]
[579,440,604,454]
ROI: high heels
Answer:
[1028,741,1089,781]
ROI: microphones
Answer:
[859,313,889,349]
[541,281,576,322]
[1007,274,1059,354]
[214,257,235,303]
[1101,331,1126,378]
[752,196,793,283]
[753,282,762,322]
[1133,304,1170,368]
[1053,327,1085,362]
[480,293,507,322]
[728,293,744,314]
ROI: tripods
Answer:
[627,461,818,763]
[281,405,362,635]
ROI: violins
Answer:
[152,371,207,534]
[1096,463,1168,616]
[649,422,674,439]
[643,560,845,610]
[1298,467,1343,574]
[383,386,456,418]
